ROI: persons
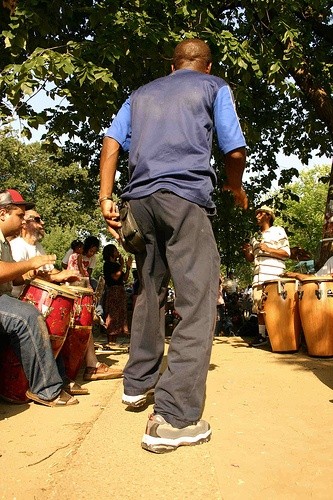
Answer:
[217,271,253,323]
[97,38,248,454]
[0,189,139,407]
[242,205,290,347]
[284,255,333,281]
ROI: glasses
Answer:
[26,217,42,222]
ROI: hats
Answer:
[254,204,275,221]
[0,189,35,211]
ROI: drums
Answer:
[61,286,94,381]
[262,278,300,353]
[299,277,333,358]
[1,278,78,404]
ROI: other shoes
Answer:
[118,333,130,338]
[107,335,116,344]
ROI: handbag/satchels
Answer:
[115,204,144,254]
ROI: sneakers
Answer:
[84,362,123,380]
[121,385,157,407]
[141,413,212,453]
[251,334,268,346]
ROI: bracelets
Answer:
[99,197,113,204]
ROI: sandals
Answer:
[26,387,78,406]
[60,376,88,394]
